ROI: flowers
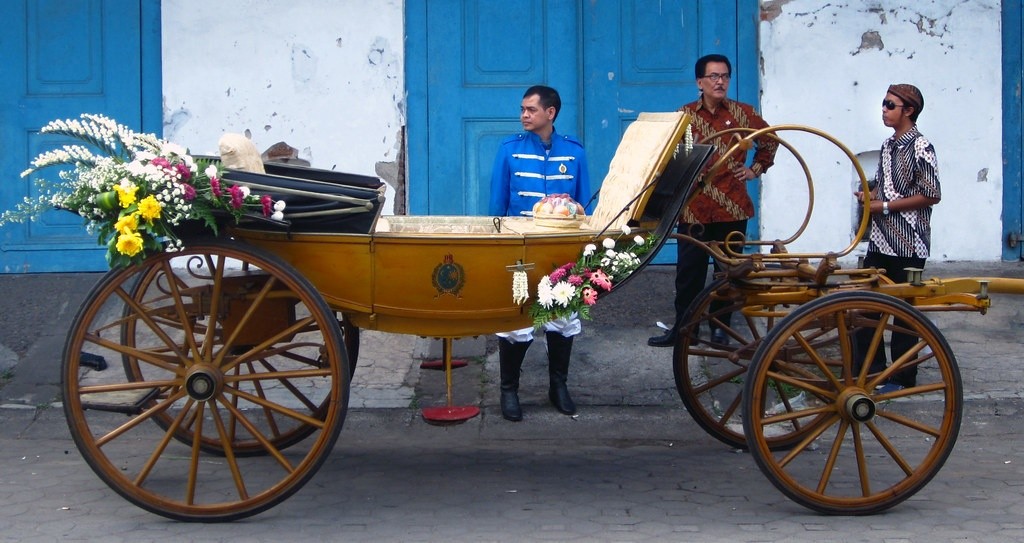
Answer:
[533,225,667,339]
[0,112,285,276]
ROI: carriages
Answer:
[61,118,1000,515]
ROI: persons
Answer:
[837,83,944,401]
[649,52,783,352]
[489,86,595,422]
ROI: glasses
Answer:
[881,99,909,111]
[700,73,731,82]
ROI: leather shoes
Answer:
[710,326,730,346]
[645,322,700,347]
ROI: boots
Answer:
[544,329,577,416]
[495,336,533,424]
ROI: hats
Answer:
[888,84,924,111]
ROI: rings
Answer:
[743,170,748,175]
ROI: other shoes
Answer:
[876,384,903,396]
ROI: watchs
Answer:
[883,201,890,215]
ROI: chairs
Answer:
[222,165,387,252]
[498,110,691,240]
